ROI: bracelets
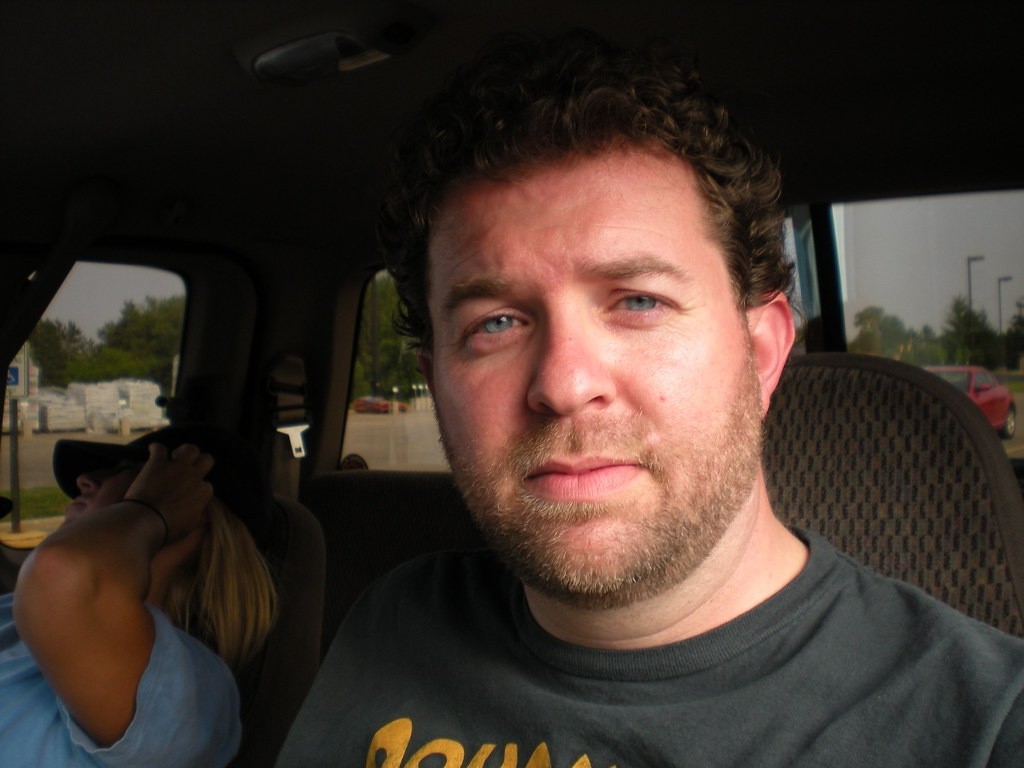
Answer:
[122,498,169,546]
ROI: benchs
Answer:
[296,470,496,669]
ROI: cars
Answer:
[355,398,407,413]
[922,365,1016,440]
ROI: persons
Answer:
[275,63,1024,768]
[0,419,272,767]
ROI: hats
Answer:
[52,422,274,543]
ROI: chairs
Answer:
[761,352,1024,639]
[226,497,327,768]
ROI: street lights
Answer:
[967,256,985,364]
[997,277,1012,374]
